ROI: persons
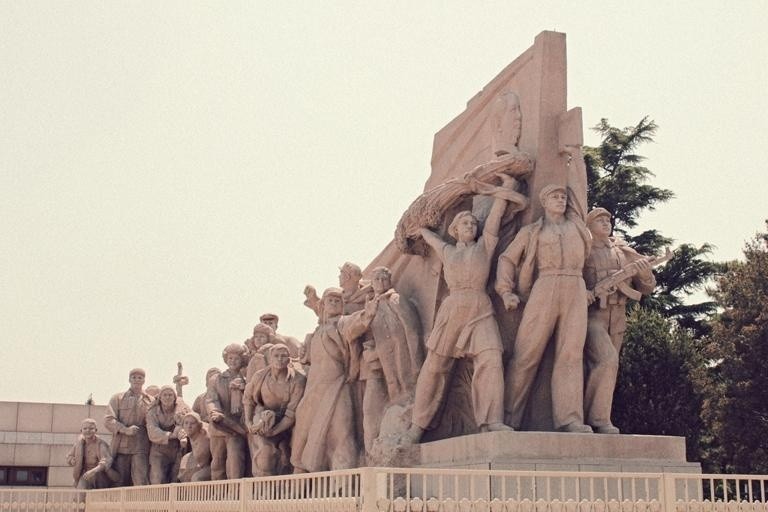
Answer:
[491,143,595,435]
[397,169,522,447]
[584,206,658,435]
[468,86,528,172]
[65,259,424,491]
